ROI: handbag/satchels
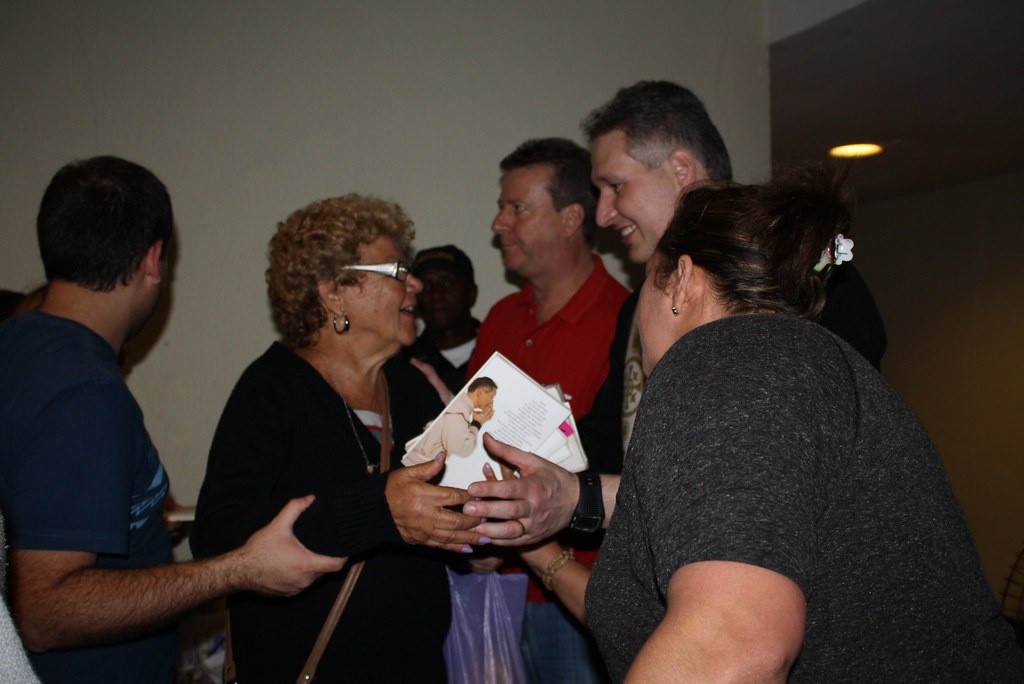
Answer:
[443,565,529,684]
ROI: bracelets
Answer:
[471,420,481,431]
[542,550,575,590]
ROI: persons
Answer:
[407,377,496,466]
[401,243,483,424]
[191,194,505,684]
[465,78,887,548]
[521,182,1024,684]
[408,137,635,682]
[1,155,348,684]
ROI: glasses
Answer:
[336,260,413,282]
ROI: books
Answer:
[165,507,196,522]
[401,352,590,493]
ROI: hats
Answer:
[408,245,475,287]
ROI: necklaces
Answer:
[314,349,380,473]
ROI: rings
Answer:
[513,520,525,536]
